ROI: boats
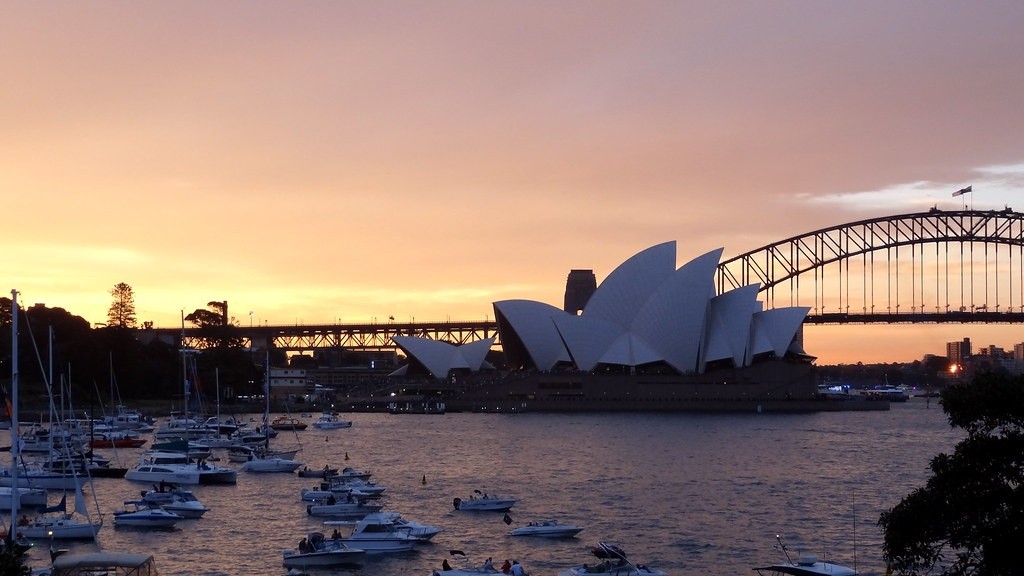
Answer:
[859,372,908,403]
[432,565,532,576]
[559,540,669,576]
[452,488,519,511]
[1,287,443,576]
[753,532,861,576]
[507,520,586,538]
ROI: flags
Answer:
[35,494,66,514]
[503,514,513,525]
[3,393,25,454]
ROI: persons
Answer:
[143,456,153,465]
[441,556,524,576]
[168,421,200,429]
[304,465,354,506]
[330,529,342,548]
[153,479,165,491]
[470,494,499,502]
[299,538,308,553]
[198,457,211,470]
[20,514,30,526]
